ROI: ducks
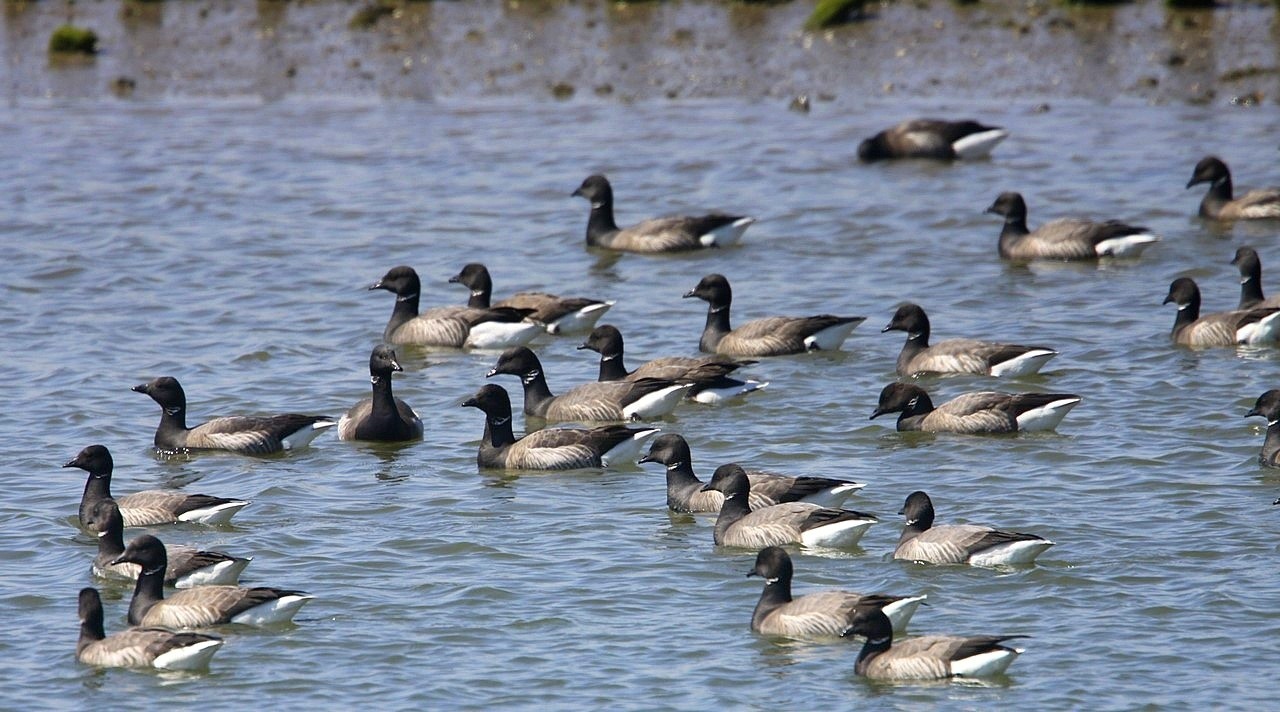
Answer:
[1272,496,1280,505]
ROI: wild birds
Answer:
[1162,245,1280,346]
[1184,152,1280,222]
[61,261,1084,682]
[569,174,756,256]
[1243,389,1279,470]
[855,117,1012,166]
[982,190,1165,261]
